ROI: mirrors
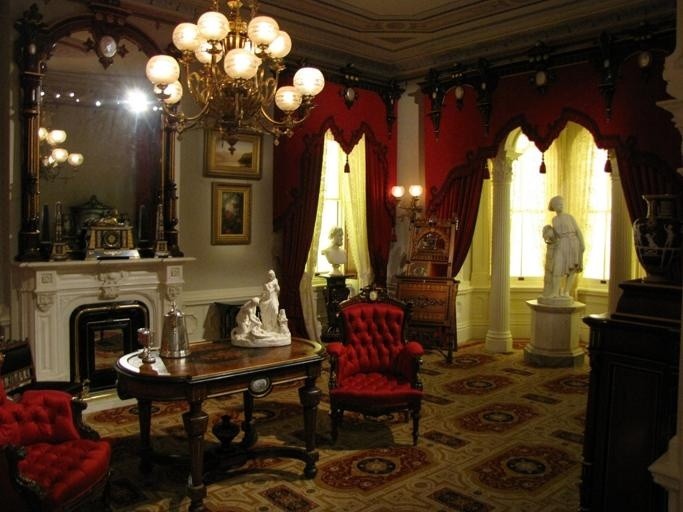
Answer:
[17,14,182,262]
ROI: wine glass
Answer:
[137,328,155,362]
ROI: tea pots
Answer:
[159,301,198,359]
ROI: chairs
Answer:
[321,278,427,451]
[1,374,113,512]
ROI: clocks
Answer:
[102,231,120,249]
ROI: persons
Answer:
[257,268,281,331]
[541,195,586,302]
[234,296,262,340]
[320,226,348,266]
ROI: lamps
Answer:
[39,125,84,179]
[144,2,337,146]
[390,184,425,212]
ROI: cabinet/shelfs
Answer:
[394,277,459,361]
[578,280,683,512]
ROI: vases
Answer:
[630,191,683,283]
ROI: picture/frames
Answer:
[201,130,263,246]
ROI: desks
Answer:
[116,338,327,512]
